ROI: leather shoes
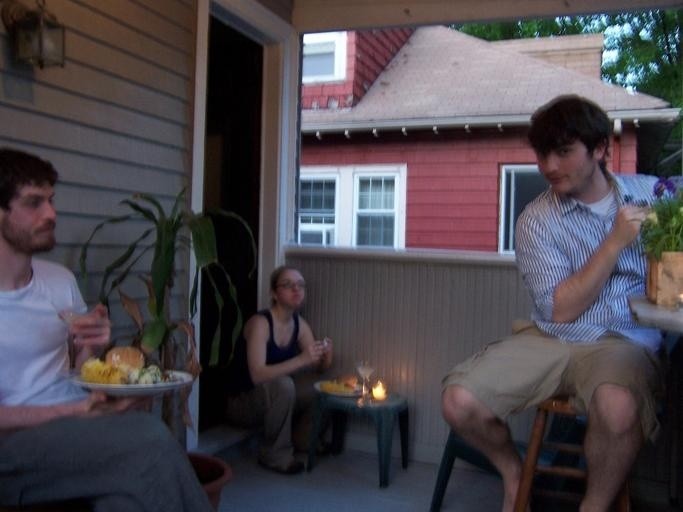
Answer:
[263,461,305,475]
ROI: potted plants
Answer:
[80,186,259,510]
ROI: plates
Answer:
[69,369,194,398]
[315,380,371,400]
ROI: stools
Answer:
[509,390,632,512]
[305,393,410,489]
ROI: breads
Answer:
[334,374,358,391]
[105,346,145,381]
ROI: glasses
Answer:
[272,281,305,290]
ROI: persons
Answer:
[0,148,216,510]
[221,264,335,473]
[436,92,674,512]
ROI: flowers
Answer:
[625,175,683,260]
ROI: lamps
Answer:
[1,1,68,70]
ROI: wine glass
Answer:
[350,360,378,403]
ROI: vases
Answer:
[646,251,683,306]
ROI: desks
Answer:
[627,292,683,336]
